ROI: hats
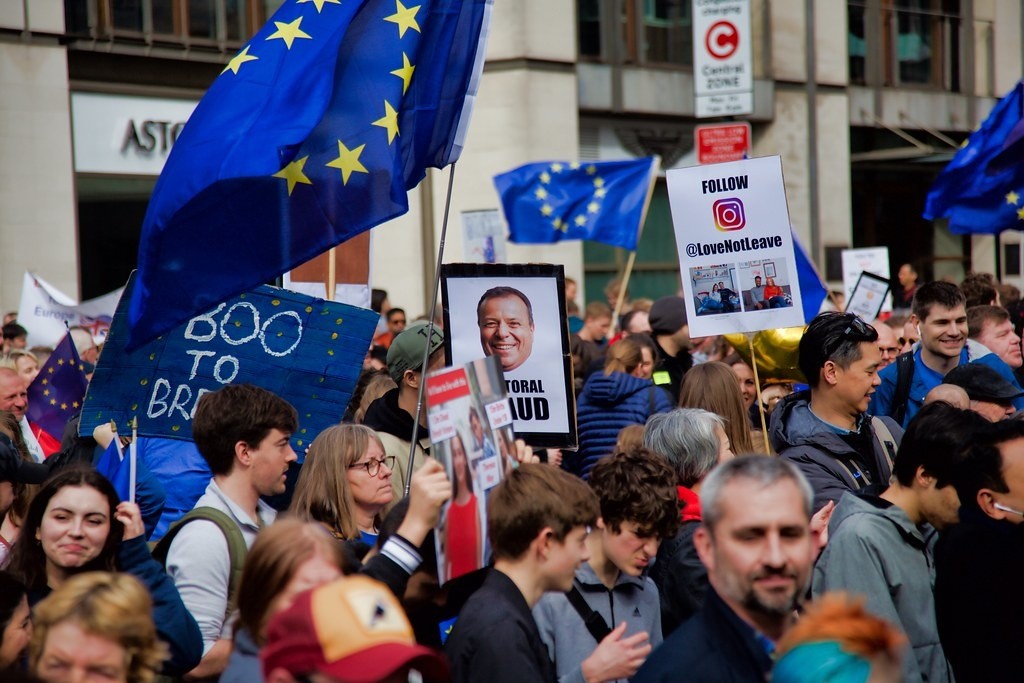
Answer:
[260,575,445,683]
[649,296,688,335]
[943,363,1024,401]
[386,323,448,381]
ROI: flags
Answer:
[923,81,1024,235]
[495,155,661,252]
[791,235,830,326]
[26,332,89,443]
[122,0,494,355]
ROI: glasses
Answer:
[348,456,396,477]
[899,337,917,346]
[393,320,407,326]
[878,347,901,358]
[823,317,867,368]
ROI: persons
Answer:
[718,282,736,313]
[750,276,768,310]
[442,431,481,582]
[0,278,1024,683]
[709,284,721,312]
[476,286,568,432]
[764,278,784,309]
[496,428,519,478]
[469,407,502,567]
